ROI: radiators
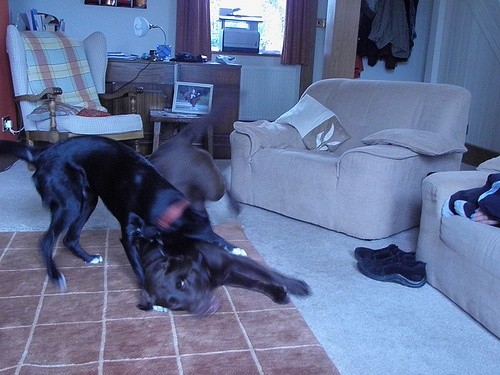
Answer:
[238,64,300,123]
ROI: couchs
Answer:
[415,155,500,343]
[230,78,471,239]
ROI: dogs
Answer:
[1,135,248,310]
[138,100,311,316]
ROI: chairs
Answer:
[5,24,145,170]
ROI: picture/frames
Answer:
[172,82,214,115]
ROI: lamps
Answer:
[132,17,167,61]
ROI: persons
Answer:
[448,172,500,229]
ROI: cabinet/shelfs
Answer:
[100,61,242,158]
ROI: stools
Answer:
[150,110,215,165]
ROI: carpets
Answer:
[0,221,340,374]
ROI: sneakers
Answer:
[353,243,416,267]
[358,254,426,288]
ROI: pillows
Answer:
[275,92,352,152]
[361,126,468,157]
[25,101,81,122]
